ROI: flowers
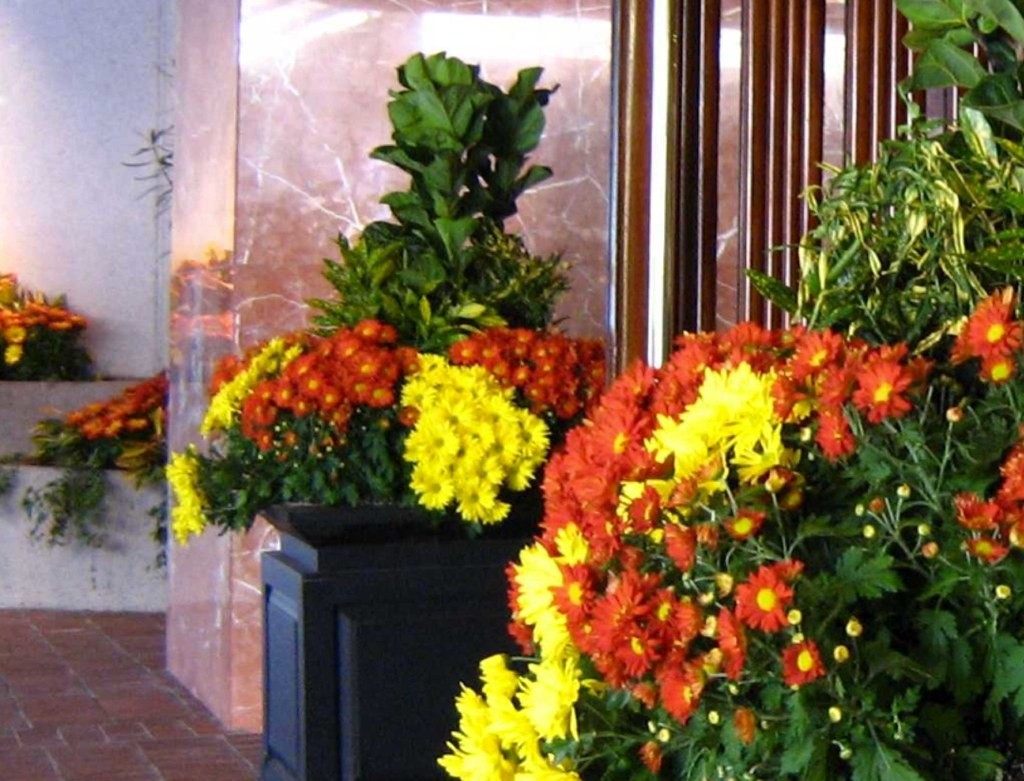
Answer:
[1,369,172,553]
[161,52,611,549]
[0,272,97,384]
